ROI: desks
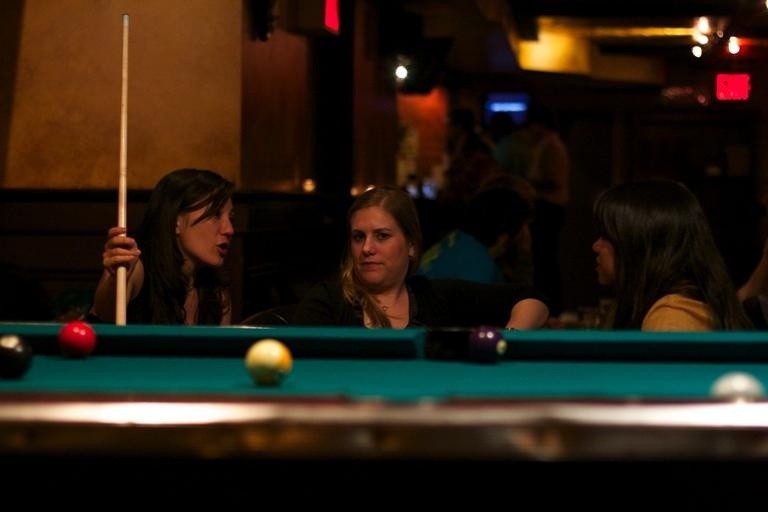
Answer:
[0,321,768,512]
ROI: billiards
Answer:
[710,372,762,402]
[469,326,508,363]
[0,335,34,379]
[245,339,294,386]
[58,322,97,358]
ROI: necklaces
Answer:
[381,304,390,312]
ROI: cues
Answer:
[114,12,129,327]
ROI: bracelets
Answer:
[503,326,520,332]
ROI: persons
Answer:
[287,183,554,360]
[79,167,240,325]
[588,176,755,332]
[397,95,584,287]
